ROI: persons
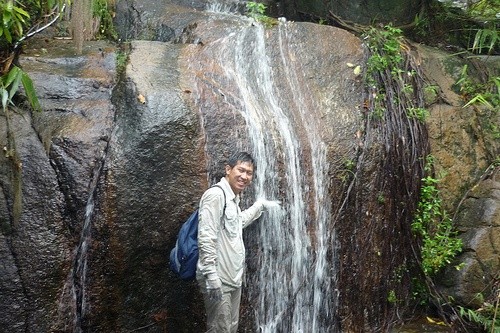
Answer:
[194,151,270,333]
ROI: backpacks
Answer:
[168,185,226,281]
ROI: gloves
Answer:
[262,199,280,211]
[205,272,224,302]
